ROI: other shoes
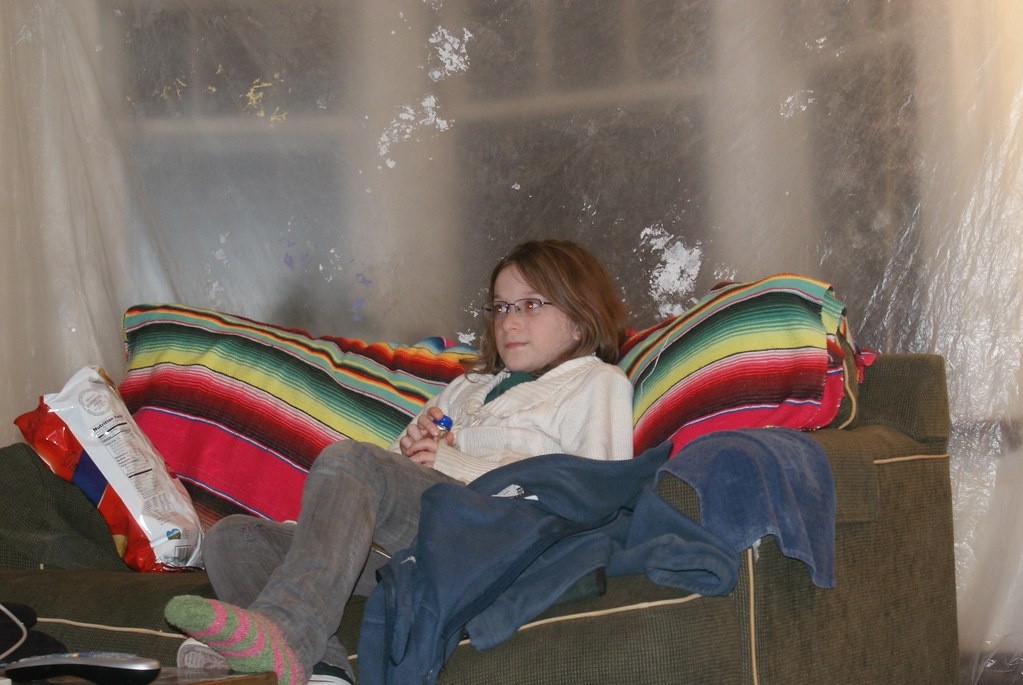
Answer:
[176,637,353,685]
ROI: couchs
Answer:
[1,273,962,684]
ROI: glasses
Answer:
[482,297,555,321]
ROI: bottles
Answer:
[424,415,454,441]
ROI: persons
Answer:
[162,239,635,685]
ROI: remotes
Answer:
[4,649,161,685]
